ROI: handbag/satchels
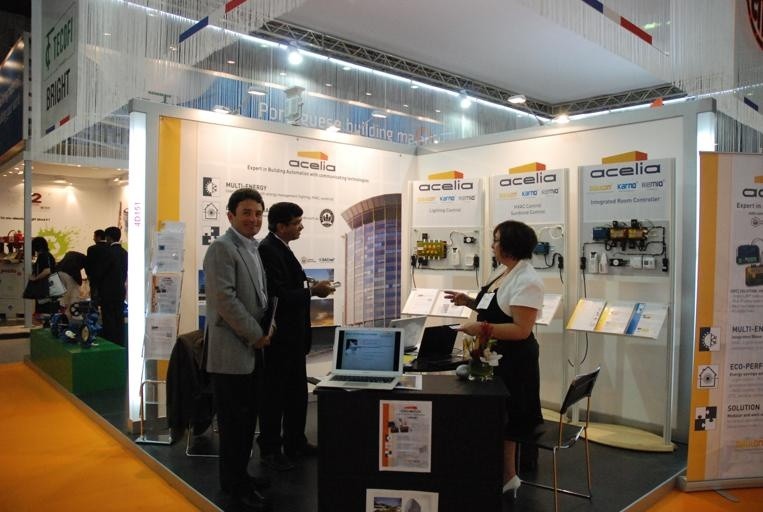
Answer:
[37,273,68,304]
[22,278,50,299]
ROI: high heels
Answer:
[502,475,521,498]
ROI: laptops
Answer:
[407,324,459,364]
[317,327,405,391]
[389,316,427,353]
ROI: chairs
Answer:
[30,328,127,394]
[165,319,253,459]
[517,367,600,511]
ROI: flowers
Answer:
[463,321,502,381]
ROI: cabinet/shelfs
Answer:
[1,257,39,317]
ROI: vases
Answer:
[471,357,490,376]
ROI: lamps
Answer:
[458,90,470,107]
[371,110,386,119]
[283,86,306,126]
[333,120,341,131]
[287,42,300,62]
[508,94,526,107]
[247,88,267,96]
[213,105,230,113]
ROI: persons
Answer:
[28,237,55,313]
[203,188,271,507]
[86,230,106,311]
[95,227,127,346]
[444,221,545,499]
[256,203,337,467]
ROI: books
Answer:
[534,293,562,324]
[401,287,469,316]
[565,295,669,338]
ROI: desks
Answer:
[314,371,519,512]
[403,348,472,371]
